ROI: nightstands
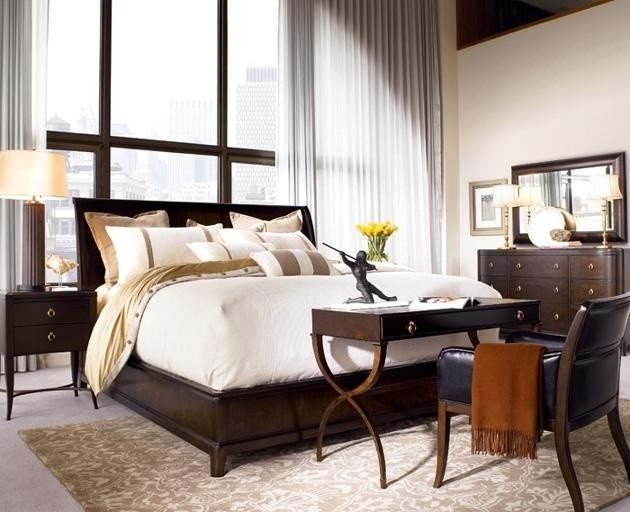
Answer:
[0,284,100,423]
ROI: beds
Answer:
[72,195,508,478]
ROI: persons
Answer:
[340,251,398,304]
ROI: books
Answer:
[408,296,481,310]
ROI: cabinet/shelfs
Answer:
[472,244,629,351]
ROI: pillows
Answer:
[82,203,342,283]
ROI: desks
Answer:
[306,295,542,488]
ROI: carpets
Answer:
[16,393,630,510]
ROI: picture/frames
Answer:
[466,176,510,236]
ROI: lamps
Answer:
[516,182,546,248]
[0,147,70,292]
[491,180,525,252]
[587,171,624,248]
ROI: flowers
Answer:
[356,219,401,265]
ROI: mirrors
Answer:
[509,149,628,248]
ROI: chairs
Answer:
[431,289,629,511]
